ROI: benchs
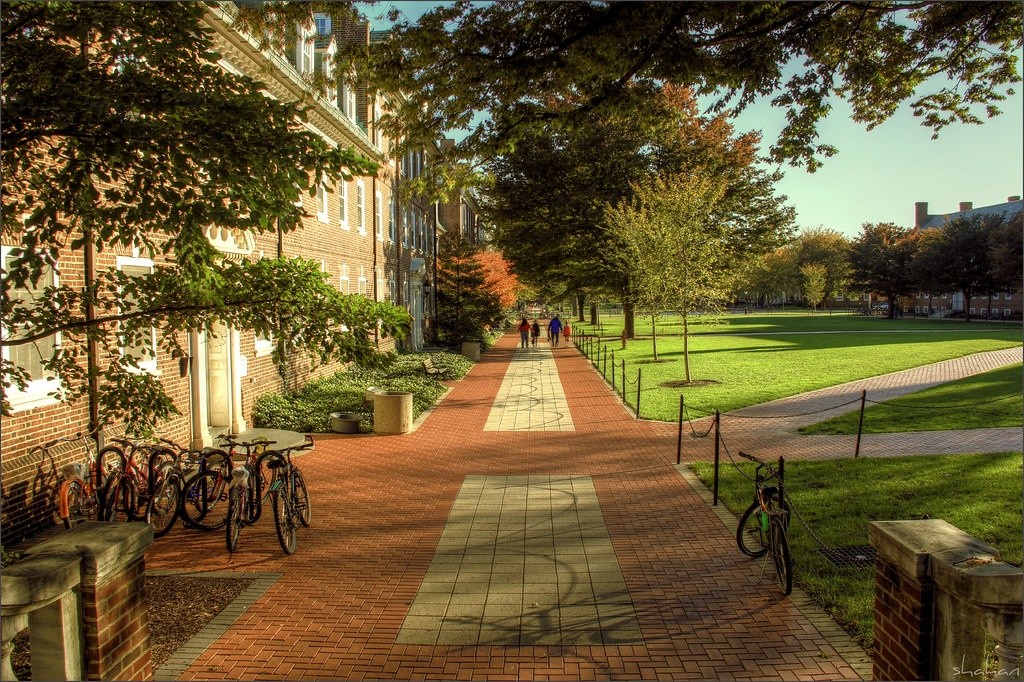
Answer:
[421,358,448,381]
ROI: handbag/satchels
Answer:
[546,335,551,341]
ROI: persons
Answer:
[563,321,571,341]
[547,316,563,348]
[531,323,541,348]
[518,318,533,349]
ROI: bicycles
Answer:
[27,417,316,560]
[735,450,798,596]
